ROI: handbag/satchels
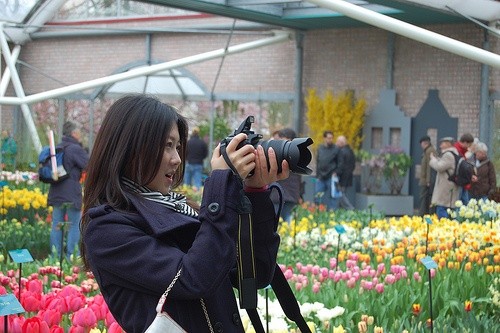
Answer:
[143,267,217,333]
[330,174,342,199]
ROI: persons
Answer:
[417,131,498,220]
[327,134,357,211]
[0,129,20,171]
[184,126,209,189]
[265,126,303,224]
[313,131,338,207]
[78,91,291,333]
[49,120,91,262]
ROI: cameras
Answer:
[220,116,313,175]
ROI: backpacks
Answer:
[443,150,473,186]
[38,142,73,186]
